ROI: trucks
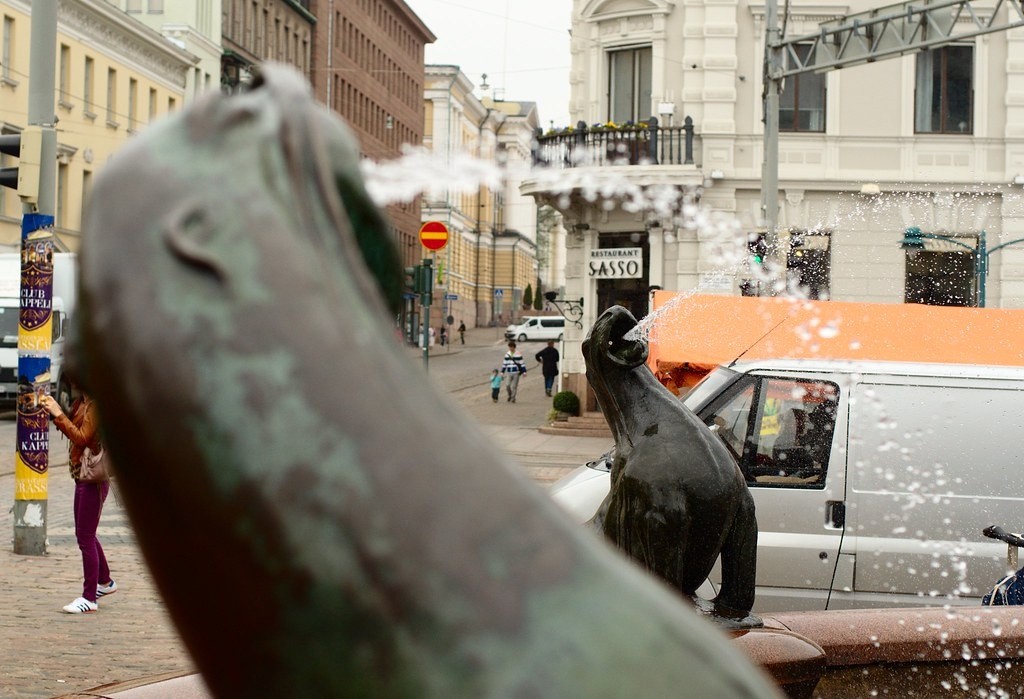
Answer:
[1,251,77,420]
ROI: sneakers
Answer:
[63,597,98,614]
[96,578,117,599]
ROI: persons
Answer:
[457,320,466,344]
[428,325,436,350]
[536,339,560,397]
[42,363,118,615]
[440,323,447,347]
[500,341,527,403]
[418,322,424,348]
[396,326,405,350]
[737,377,840,484]
[490,368,504,403]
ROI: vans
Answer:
[542,357,1024,612]
[504,316,565,342]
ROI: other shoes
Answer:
[545,390,552,398]
[511,399,515,402]
[507,397,511,401]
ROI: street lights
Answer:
[895,227,988,307]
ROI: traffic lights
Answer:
[404,266,420,292]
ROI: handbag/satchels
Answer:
[517,364,523,376]
[443,331,448,337]
[979,533,1024,606]
[78,444,109,482]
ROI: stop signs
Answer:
[418,221,449,252]
